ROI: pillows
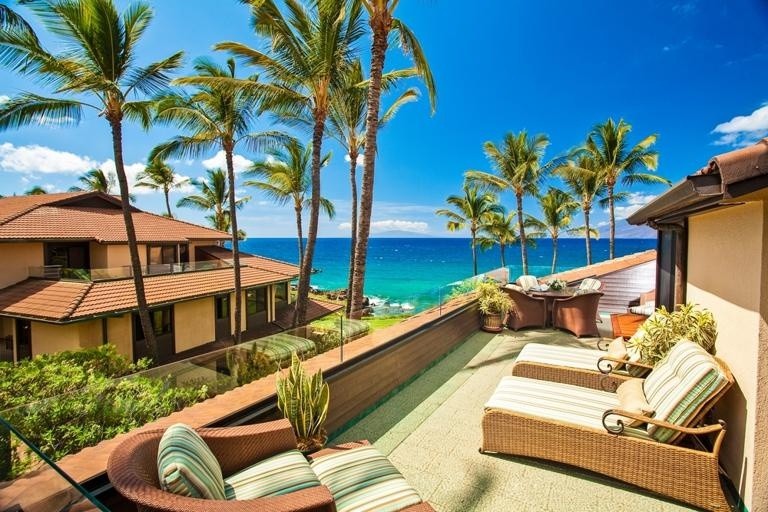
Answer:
[608,335,628,369]
[616,378,654,428]
[155,422,225,502]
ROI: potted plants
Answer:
[276,348,331,456]
[474,277,519,332]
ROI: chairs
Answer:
[477,356,738,508]
[104,418,438,511]
[512,310,718,393]
[502,273,655,340]
